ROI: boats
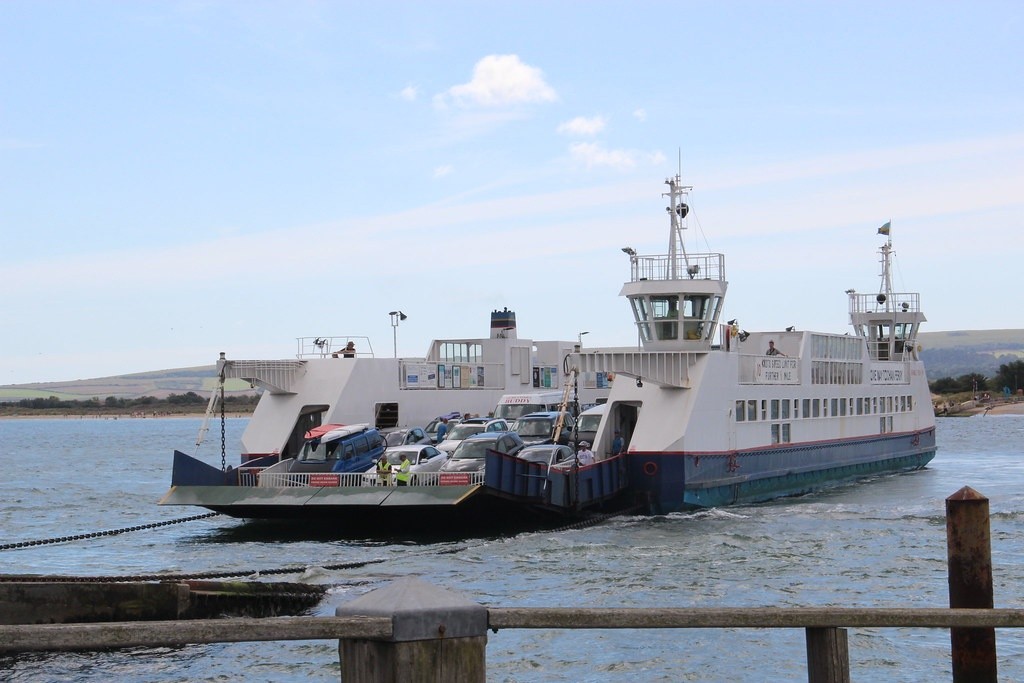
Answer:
[154,145,937,531]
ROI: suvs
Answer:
[422,411,574,459]
[436,429,525,485]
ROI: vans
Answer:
[567,402,608,452]
[492,389,582,429]
[283,424,386,487]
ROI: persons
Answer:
[332,341,356,357]
[765,340,789,357]
[576,441,596,468]
[394,455,410,486]
[610,428,625,457]
[460,413,470,424]
[376,455,392,486]
[437,418,449,442]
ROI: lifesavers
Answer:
[644,462,658,476]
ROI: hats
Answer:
[399,455,406,459]
[381,454,387,460]
[615,429,620,433]
[347,341,355,346]
[579,441,589,446]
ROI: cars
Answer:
[516,443,574,468]
[361,444,449,486]
[382,426,432,447]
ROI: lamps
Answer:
[728,319,749,342]
[786,326,793,331]
